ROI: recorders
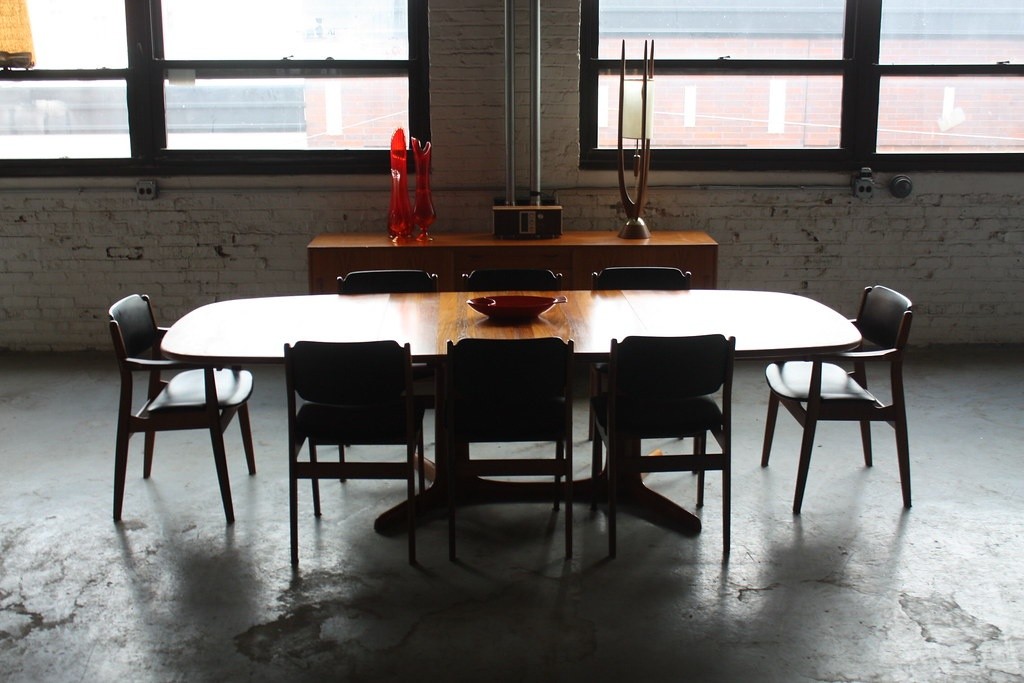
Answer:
[492,206,563,239]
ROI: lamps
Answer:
[618,38,658,241]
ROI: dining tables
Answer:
[161,288,863,537]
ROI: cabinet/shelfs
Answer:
[306,230,721,294]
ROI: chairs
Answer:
[337,268,440,484]
[283,340,428,568]
[434,336,575,560]
[761,284,913,516]
[590,333,735,554]
[461,267,562,292]
[580,266,692,439]
[107,293,256,525]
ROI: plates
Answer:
[467,295,567,319]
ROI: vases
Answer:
[389,126,413,243]
[411,137,435,243]
